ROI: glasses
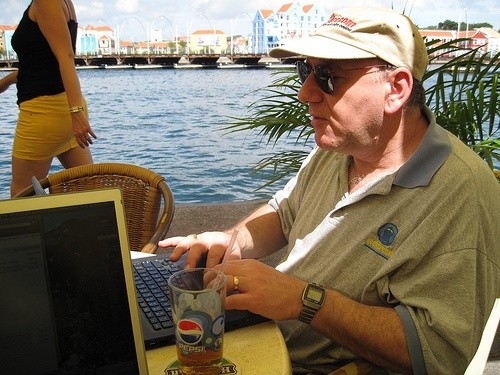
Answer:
[294,60,396,95]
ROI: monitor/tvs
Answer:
[0,188,150,375]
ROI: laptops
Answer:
[131,252,273,347]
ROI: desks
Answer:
[131,252,290,375]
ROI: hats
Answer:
[268,7,429,82]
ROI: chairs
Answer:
[11,163,174,254]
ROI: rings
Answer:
[191,233,198,240]
[233,275,239,289]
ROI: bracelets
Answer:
[69,106,84,113]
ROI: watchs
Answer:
[298,282,327,326]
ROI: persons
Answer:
[158,5,500,375]
[0,0,97,200]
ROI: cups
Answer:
[168,268,226,375]
